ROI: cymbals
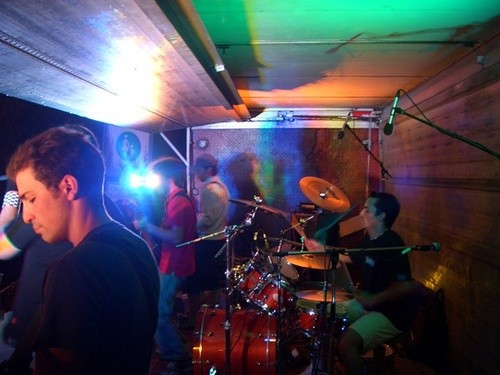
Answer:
[298,175,351,213]
[284,249,342,269]
[228,197,287,217]
[296,288,349,303]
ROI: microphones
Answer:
[338,112,351,139]
[402,241,442,254]
[384,89,400,135]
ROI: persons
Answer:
[322,191,414,375]
[132,158,198,375]
[176,150,232,325]
[1,127,161,375]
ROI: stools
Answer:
[363,334,404,375]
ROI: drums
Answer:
[295,298,348,340]
[226,247,300,320]
[192,308,282,375]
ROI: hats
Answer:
[189,154,217,170]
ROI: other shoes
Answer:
[159,360,193,375]
[176,315,194,330]
